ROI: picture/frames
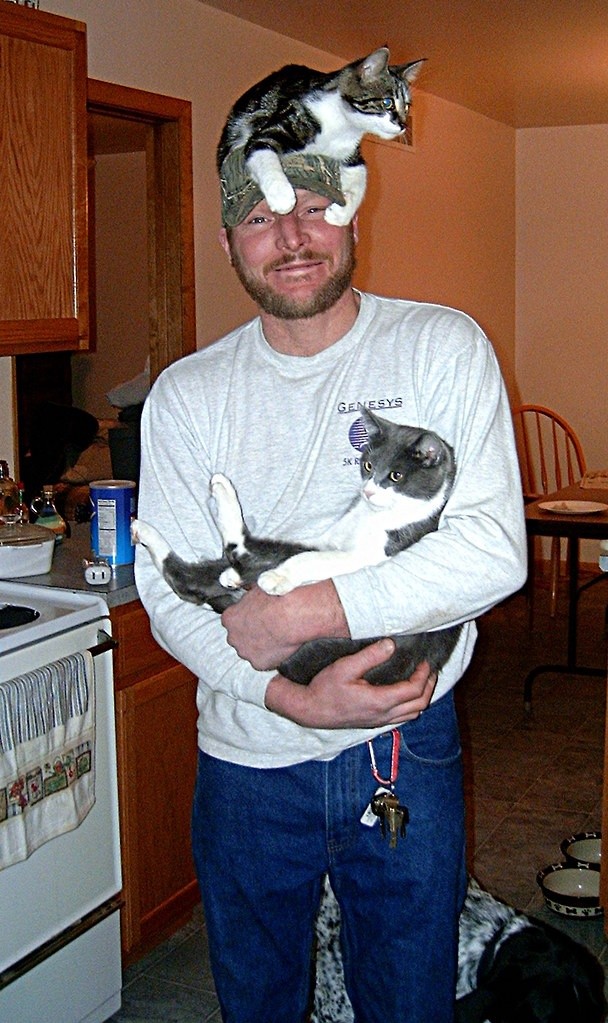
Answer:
[362,110,416,155]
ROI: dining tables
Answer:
[519,469,608,719]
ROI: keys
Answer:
[372,792,410,850]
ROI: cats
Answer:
[130,401,466,686]
[216,41,428,226]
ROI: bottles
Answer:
[0,459,28,523]
[30,485,66,545]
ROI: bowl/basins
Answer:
[536,863,603,917]
[560,831,602,863]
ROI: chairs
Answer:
[511,404,589,620]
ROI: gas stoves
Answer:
[0,579,113,655]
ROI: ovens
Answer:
[0,619,121,1023]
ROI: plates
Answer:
[538,501,608,514]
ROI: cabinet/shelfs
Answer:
[105,598,204,967]
[0,0,90,357]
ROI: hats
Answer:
[220,146,346,227]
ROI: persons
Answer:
[134,146,529,1023]
